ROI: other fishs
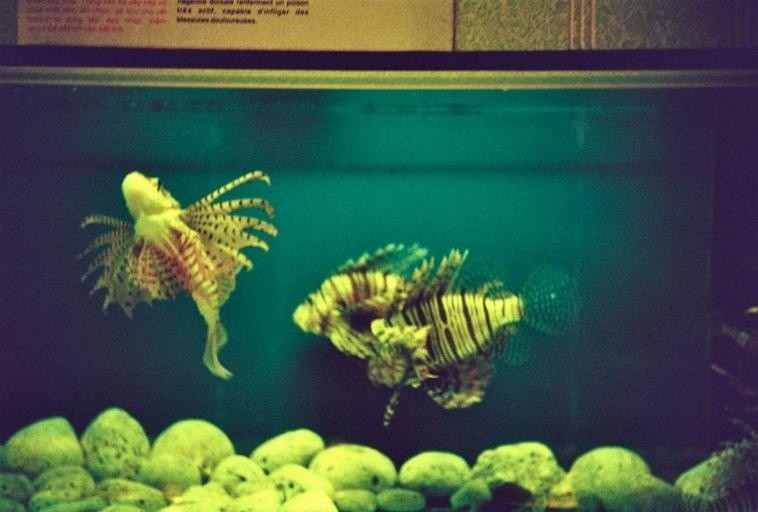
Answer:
[66,167,278,383]
[292,240,585,429]
